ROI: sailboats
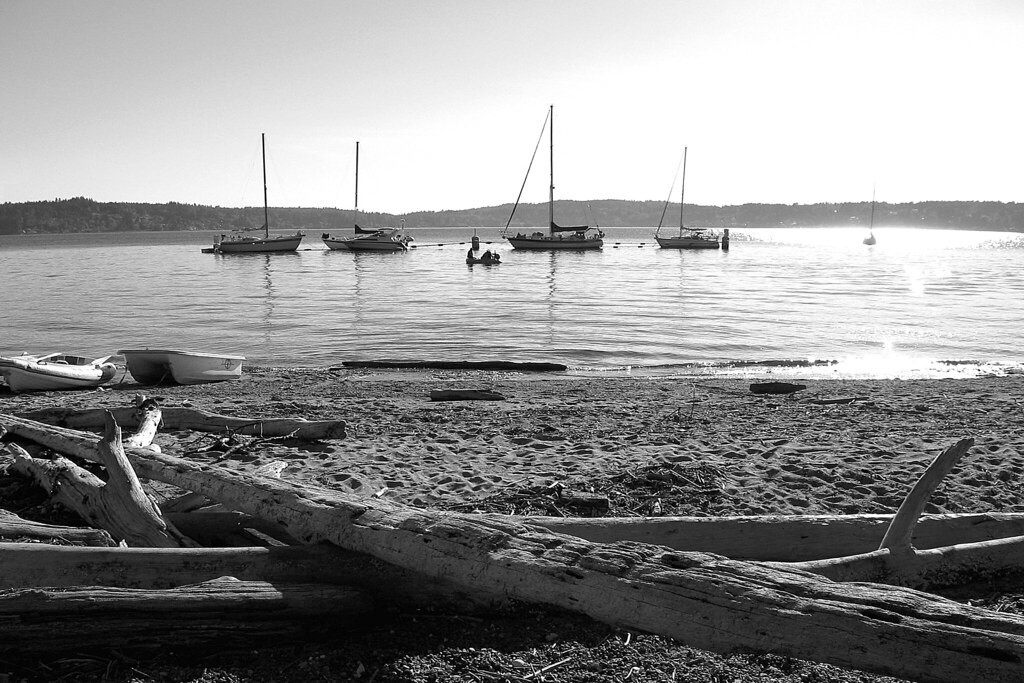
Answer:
[502,104,606,249]
[319,140,415,251]
[200,132,303,253]
[654,146,720,249]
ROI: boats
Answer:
[0,353,117,394]
[117,347,246,382]
